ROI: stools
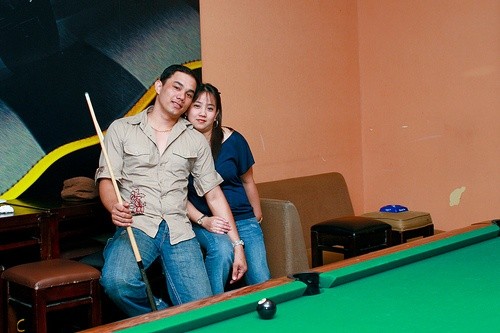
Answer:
[311,216,392,269]
[360,210,434,244]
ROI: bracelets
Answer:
[232,240,244,248]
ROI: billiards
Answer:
[256,298,276,319]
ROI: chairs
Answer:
[0,202,118,333]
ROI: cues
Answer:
[83,92,156,312]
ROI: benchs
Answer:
[253,172,356,279]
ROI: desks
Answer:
[72,219,500,333]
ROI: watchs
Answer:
[196,215,206,225]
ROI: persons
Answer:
[95,65,248,317]
[187,83,270,296]
